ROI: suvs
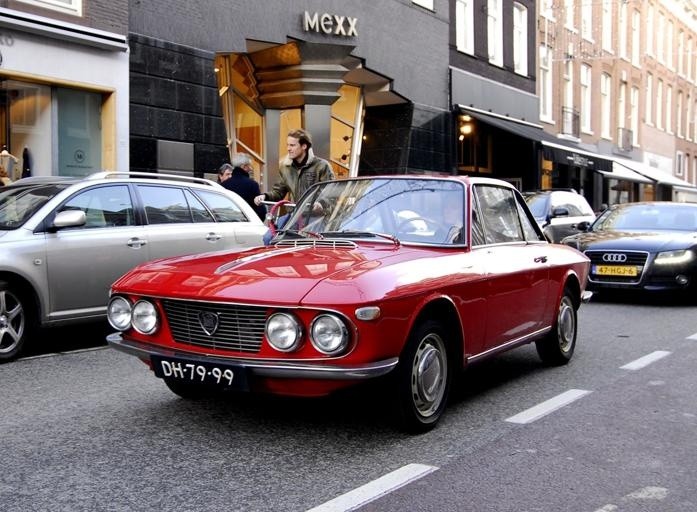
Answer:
[515,188,595,244]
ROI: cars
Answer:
[102,172,595,433]
[0,170,279,363]
[559,200,697,302]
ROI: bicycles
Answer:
[258,198,320,244]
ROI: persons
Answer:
[221,151,268,222]
[217,163,234,184]
[254,129,335,246]
[434,192,463,238]
